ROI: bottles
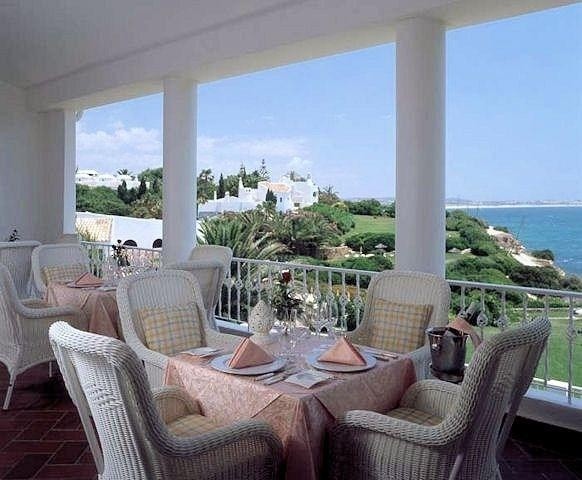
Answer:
[445,301,478,336]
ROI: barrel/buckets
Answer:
[425,326,469,372]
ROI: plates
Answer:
[304,349,377,374]
[66,281,102,288]
[211,353,287,376]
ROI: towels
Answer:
[74,270,104,284]
[229,337,274,368]
[318,336,368,366]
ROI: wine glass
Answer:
[308,301,339,343]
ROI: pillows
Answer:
[371,294,433,358]
[42,265,88,287]
[139,300,203,352]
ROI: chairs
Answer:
[1,239,41,297]
[327,316,553,480]
[33,245,234,340]
[344,269,452,382]
[116,270,246,391]
[0,263,88,411]
[46,320,284,480]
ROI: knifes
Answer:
[264,368,301,386]
[364,349,399,361]
